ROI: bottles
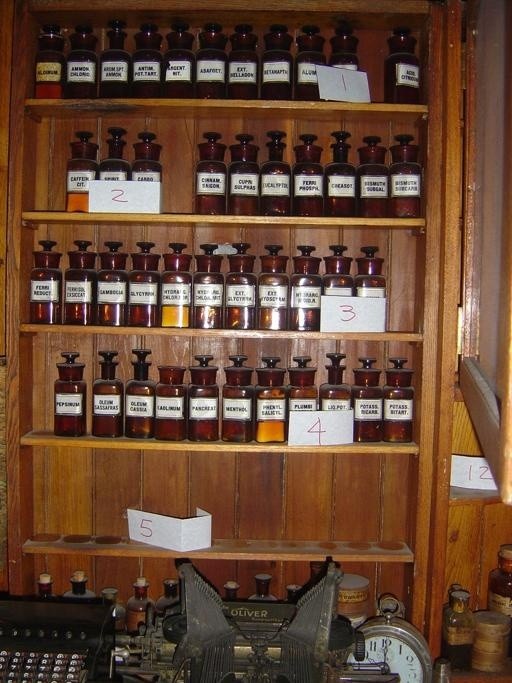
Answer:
[356,244,385,297]
[35,566,301,630]
[27,238,64,327]
[53,350,87,438]
[64,239,97,327]
[488,544,511,657]
[303,560,323,592]
[328,23,358,70]
[287,356,317,411]
[258,21,293,101]
[195,22,226,102]
[125,348,154,439]
[319,351,352,410]
[295,24,326,101]
[288,246,319,331]
[443,590,473,668]
[228,21,258,102]
[320,243,354,296]
[221,354,253,443]
[134,21,162,101]
[161,20,195,101]
[155,364,186,442]
[255,356,286,445]
[158,242,193,329]
[129,238,158,327]
[65,21,99,103]
[38,21,65,101]
[193,128,422,217]
[90,351,123,438]
[193,243,223,329]
[383,24,420,103]
[433,659,450,683]
[384,357,412,444]
[95,237,128,328]
[100,21,132,101]
[226,241,257,330]
[187,355,220,443]
[66,126,163,213]
[352,356,385,443]
[257,245,287,331]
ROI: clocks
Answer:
[347,593,434,683]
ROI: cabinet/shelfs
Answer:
[439,0,512,683]
[0,0,442,640]
